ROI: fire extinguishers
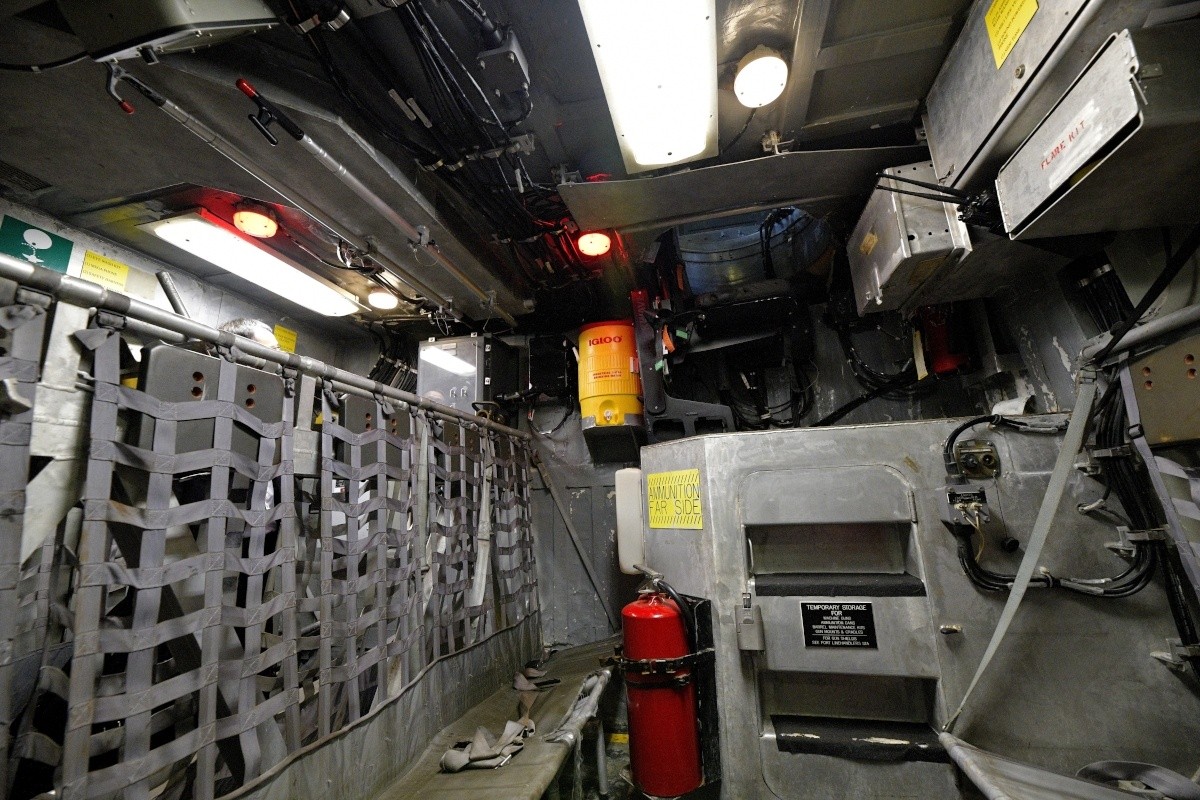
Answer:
[615,566,705,798]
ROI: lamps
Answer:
[366,284,400,310]
[233,202,280,239]
[733,43,789,110]
